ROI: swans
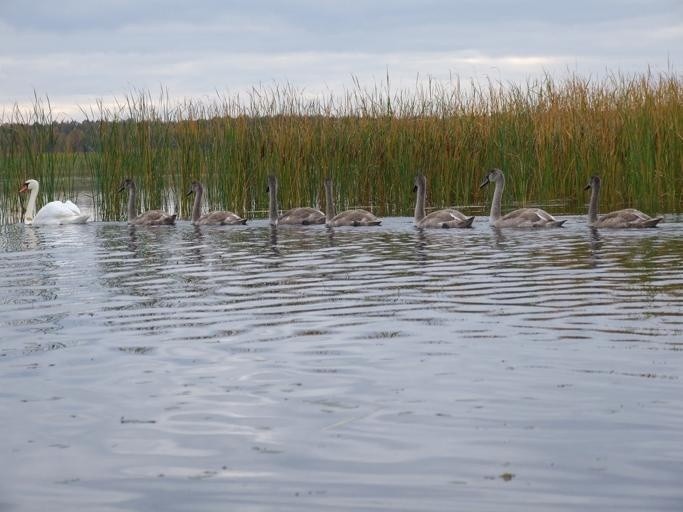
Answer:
[18,178,91,225]
[581,175,665,227]
[117,178,176,225]
[186,180,248,225]
[264,174,326,225]
[322,178,384,226]
[478,168,568,228]
[411,171,475,229]
